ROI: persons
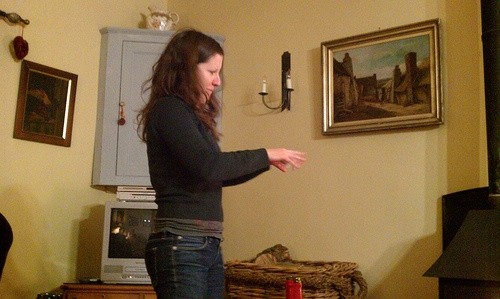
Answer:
[134,27,308,299]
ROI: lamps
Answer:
[258,51,294,112]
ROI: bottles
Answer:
[285,277,302,299]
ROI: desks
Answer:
[59,282,159,299]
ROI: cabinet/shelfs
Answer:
[91,26,227,185]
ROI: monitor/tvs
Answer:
[100,200,159,284]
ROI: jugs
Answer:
[146,6,180,31]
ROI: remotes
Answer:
[78,278,99,284]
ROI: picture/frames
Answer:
[320,18,445,136]
[13,59,79,147]
